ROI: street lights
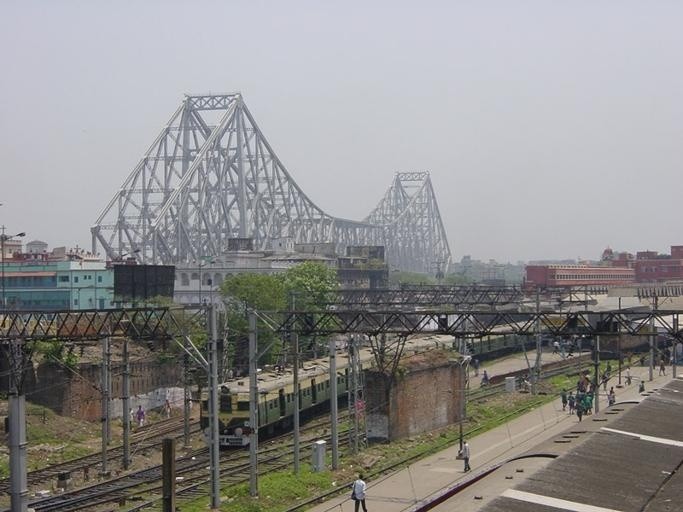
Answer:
[120,249,140,262]
[1,232,27,311]
[198,260,216,316]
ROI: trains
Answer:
[195,315,558,450]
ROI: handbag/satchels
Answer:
[351,491,356,500]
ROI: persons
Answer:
[553,334,683,422]
[479,370,488,388]
[353,393,366,429]
[352,475,368,512]
[136,404,146,428]
[166,399,171,419]
[129,407,134,431]
[459,439,471,472]
[472,356,480,377]
